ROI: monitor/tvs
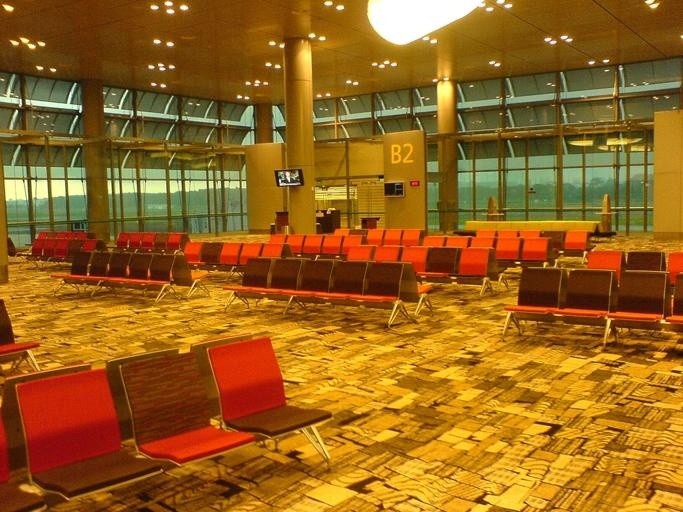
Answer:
[384,181,404,197]
[273,168,304,187]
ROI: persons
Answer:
[283,171,297,184]
